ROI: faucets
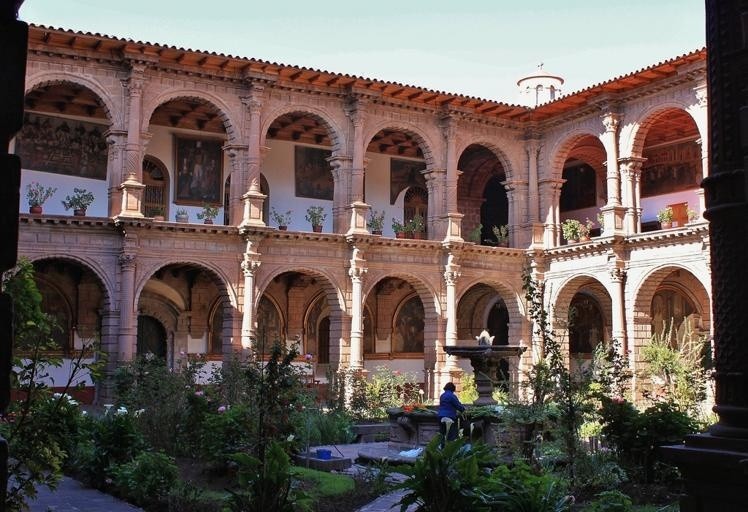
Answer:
[304,415,314,469]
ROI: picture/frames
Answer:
[174,132,223,208]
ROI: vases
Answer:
[413,231,421,239]
[279,225,287,230]
[30,206,42,213]
[580,236,588,242]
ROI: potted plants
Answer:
[561,219,581,244]
[469,224,509,247]
[151,201,220,224]
[656,207,674,229]
[366,206,407,238]
[685,208,697,223]
[305,205,328,232]
[61,187,94,216]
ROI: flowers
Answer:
[579,217,595,240]
[24,179,58,207]
[405,215,426,234]
[269,205,296,225]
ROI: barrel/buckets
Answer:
[589,435,599,454]
[316,451,333,459]
[601,433,608,447]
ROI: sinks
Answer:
[295,450,353,474]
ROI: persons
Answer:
[436,382,467,451]
[185,140,214,198]
